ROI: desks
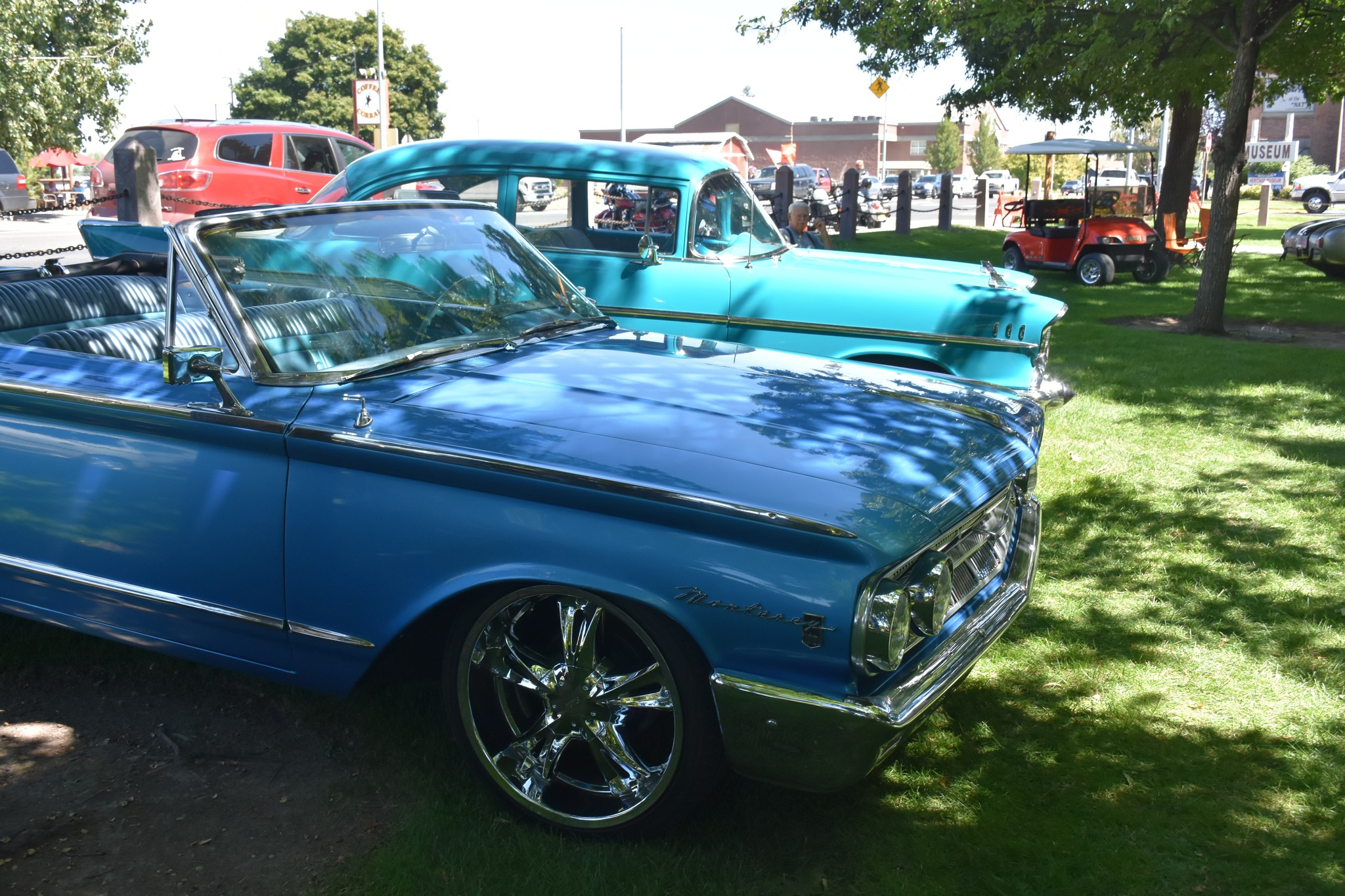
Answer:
[53,191,83,210]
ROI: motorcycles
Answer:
[803,178,891,231]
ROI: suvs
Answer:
[912,173,942,199]
[952,174,973,198]
[747,163,818,200]
[89,114,437,226]
[1288,165,1345,214]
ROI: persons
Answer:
[45,181,60,203]
[748,166,757,179]
[72,181,86,202]
[1190,173,1213,201]
[855,160,870,198]
[778,202,834,251]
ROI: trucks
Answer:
[436,172,555,212]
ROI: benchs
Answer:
[523,227,598,250]
[1025,199,1092,238]
[0,275,403,373]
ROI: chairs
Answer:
[1162,208,1252,273]
[35,182,57,208]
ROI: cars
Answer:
[1280,217,1345,275]
[1061,180,1084,195]
[880,175,900,196]
[0,201,1047,843]
[77,138,1074,427]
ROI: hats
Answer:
[74,181,81,185]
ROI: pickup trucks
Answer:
[973,170,1019,198]
[1089,168,1142,188]
[813,167,845,201]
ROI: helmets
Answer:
[605,182,627,196]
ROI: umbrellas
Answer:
[28,144,98,179]
